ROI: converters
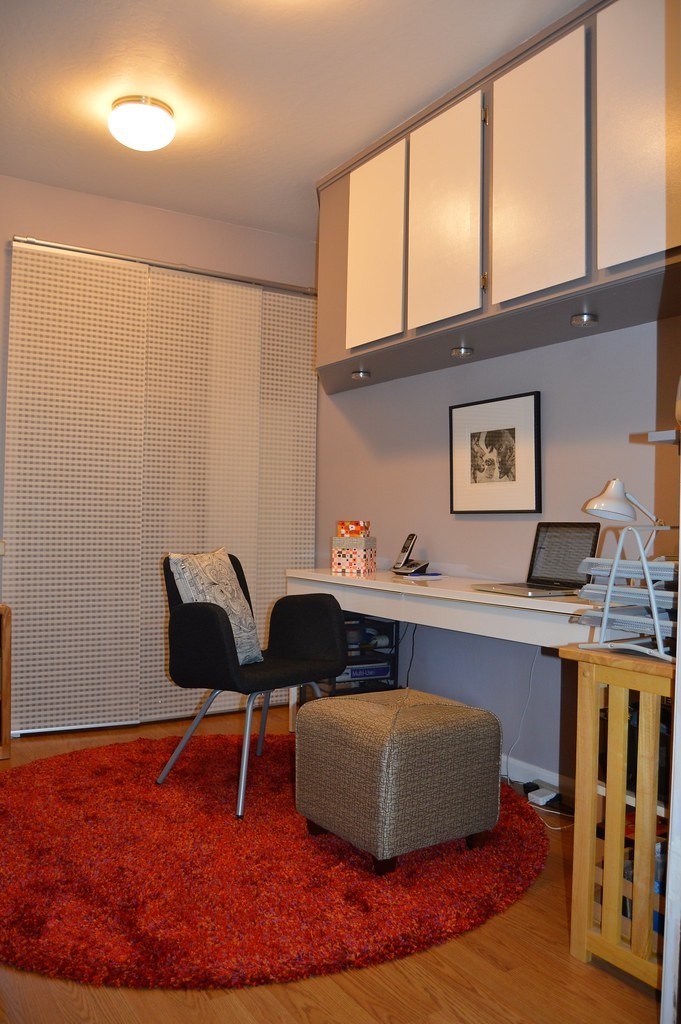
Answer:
[528,788,557,806]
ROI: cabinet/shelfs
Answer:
[558,636,676,992]
[314,0,681,395]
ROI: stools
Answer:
[294,689,504,878]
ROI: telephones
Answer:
[389,533,430,576]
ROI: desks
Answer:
[284,567,627,850]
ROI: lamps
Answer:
[107,94,179,152]
[585,477,665,589]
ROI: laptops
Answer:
[471,521,601,596]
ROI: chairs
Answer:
[154,547,348,818]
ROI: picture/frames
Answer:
[449,389,543,516]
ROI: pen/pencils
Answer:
[409,573,442,576]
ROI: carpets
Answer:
[0,733,549,989]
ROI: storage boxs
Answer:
[332,537,376,573]
[336,520,371,538]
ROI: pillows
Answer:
[166,546,265,665]
